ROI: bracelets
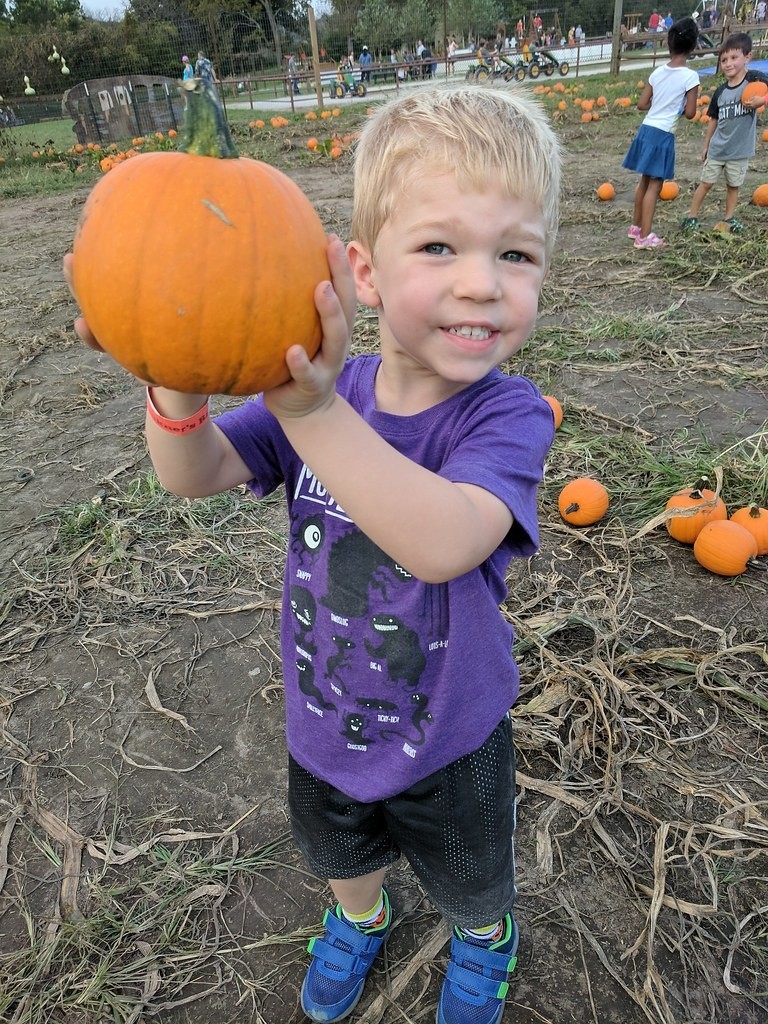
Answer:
[146,384,211,435]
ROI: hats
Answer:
[363,46,368,50]
[182,56,188,61]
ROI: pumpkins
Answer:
[538,394,768,576]
[531,82,768,208]
[73,76,330,396]
[30,105,381,173]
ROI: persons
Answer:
[471,15,582,81]
[62,84,562,1024]
[446,38,459,78]
[638,22,648,32]
[664,13,673,31]
[359,46,371,83]
[657,14,665,48]
[622,16,701,249]
[289,53,301,95]
[756,0,767,24]
[341,51,354,69]
[181,51,219,110]
[692,9,699,25]
[0,96,16,126]
[721,6,731,43]
[390,39,437,83]
[702,8,717,41]
[321,48,325,63]
[646,8,659,50]
[681,34,768,235]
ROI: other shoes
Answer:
[680,217,710,242]
[722,217,744,233]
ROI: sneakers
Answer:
[634,232,667,250]
[436,909,519,1024]
[300,882,392,1024]
[628,225,641,238]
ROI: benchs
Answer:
[373,69,417,84]
